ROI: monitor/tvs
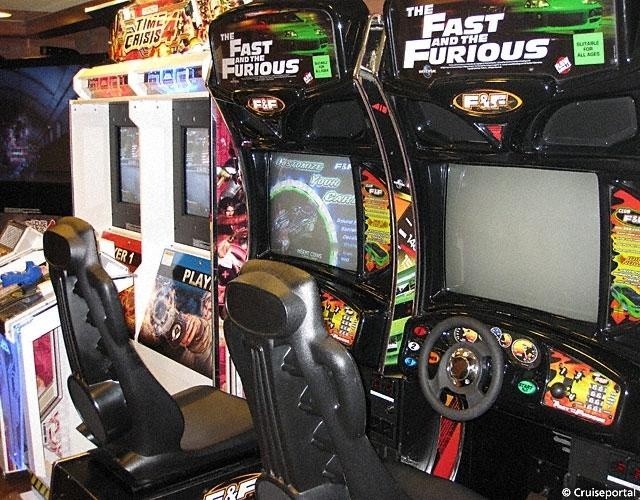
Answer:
[172,98,211,251]
[109,101,140,233]
[428,162,605,329]
[260,149,362,273]
[0,52,107,216]
